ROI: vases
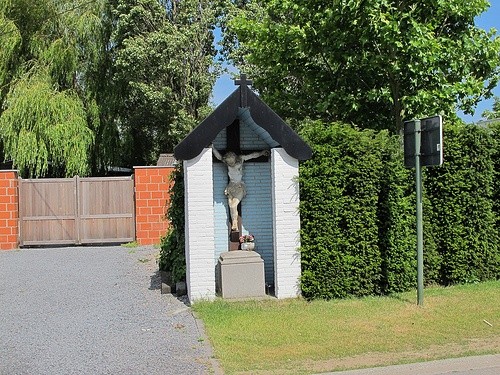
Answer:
[241,243,254,250]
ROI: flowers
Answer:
[239,234,254,243]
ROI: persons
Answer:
[210,145,269,229]
